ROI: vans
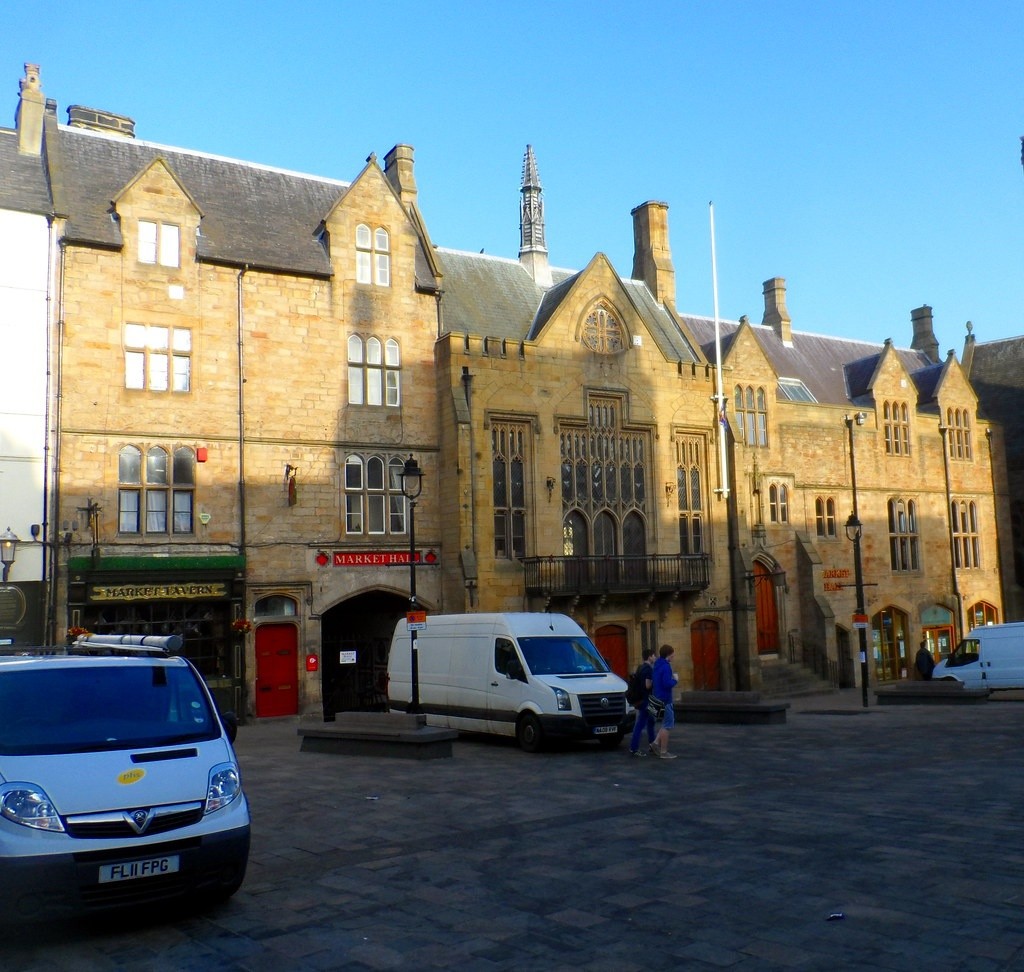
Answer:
[930,621,1024,699]
[0,655,252,926]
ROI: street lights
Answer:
[845,509,872,704]
[397,454,425,713]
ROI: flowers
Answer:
[66,625,88,644]
[230,618,252,633]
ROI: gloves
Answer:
[673,674,678,681]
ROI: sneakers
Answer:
[660,751,677,759]
[629,748,647,757]
[650,741,661,756]
[648,746,651,751]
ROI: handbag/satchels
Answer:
[647,695,665,719]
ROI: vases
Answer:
[234,631,244,640]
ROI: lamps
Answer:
[0,526,21,582]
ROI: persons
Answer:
[628,649,657,756]
[916,642,936,680]
[649,644,679,759]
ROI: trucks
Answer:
[382,614,636,751]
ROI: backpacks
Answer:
[626,664,650,708]
